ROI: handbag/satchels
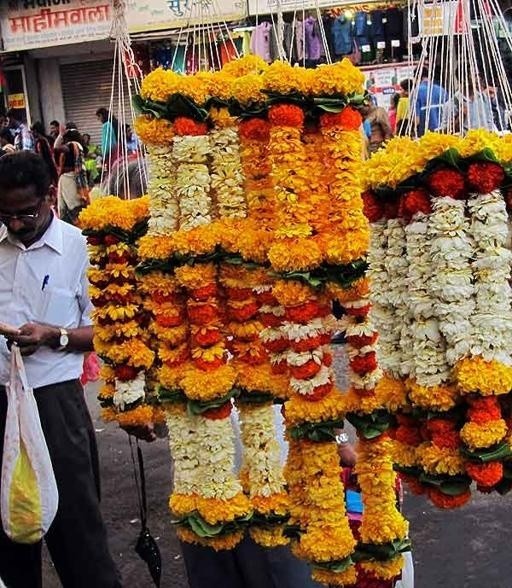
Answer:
[0,344,59,543]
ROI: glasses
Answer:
[0,197,44,223]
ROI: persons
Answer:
[106,155,359,587]
[0,149,124,588]
[359,51,512,161]
[333,412,414,586]
[0,105,141,225]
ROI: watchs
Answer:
[54,325,70,353]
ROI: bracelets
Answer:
[334,432,350,446]
